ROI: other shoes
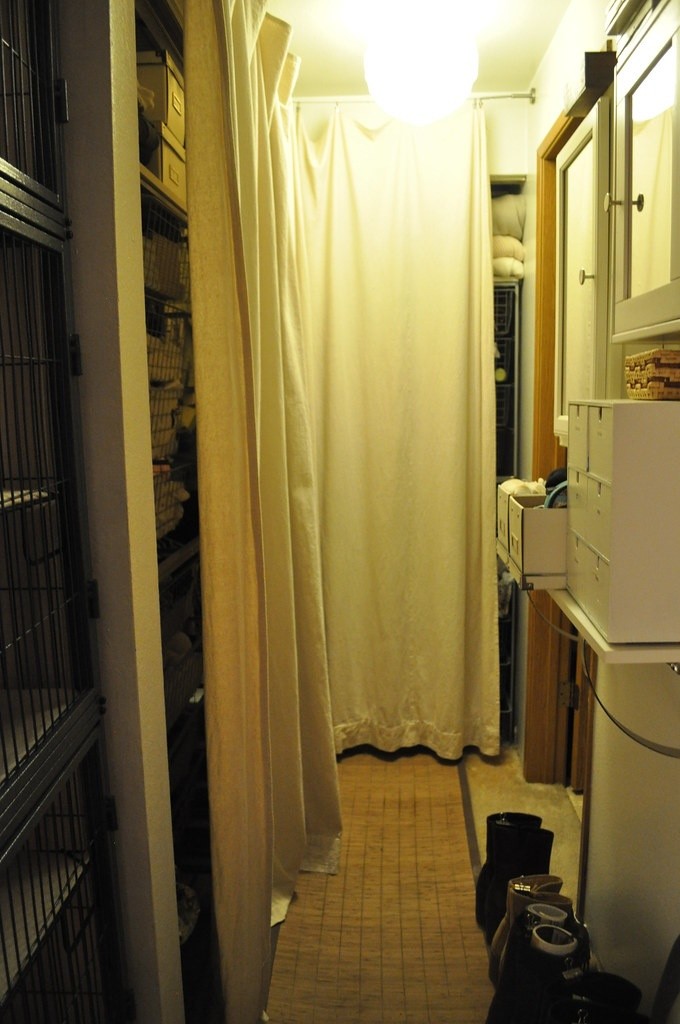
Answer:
[490,873,572,979]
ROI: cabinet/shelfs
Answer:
[60,2,235,1024]
[496,24,679,1024]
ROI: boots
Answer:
[474,812,554,943]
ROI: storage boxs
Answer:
[135,50,190,207]
